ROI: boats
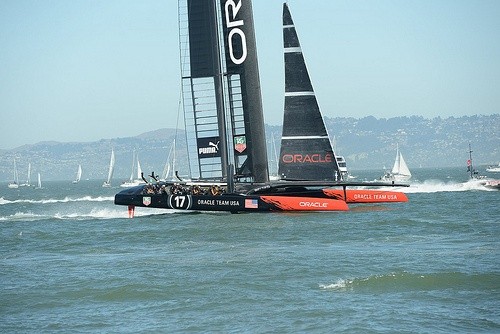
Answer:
[486,165,500,173]
[464,143,500,188]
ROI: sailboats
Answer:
[102,148,115,187]
[362,144,412,182]
[20,162,33,187]
[120,148,139,187]
[7,159,20,188]
[134,161,147,184]
[114,0,353,211]
[246,4,410,204]
[156,140,177,181]
